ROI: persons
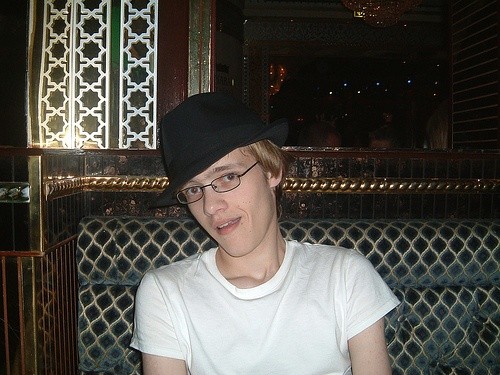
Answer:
[297,122,342,147]
[129,90,403,374]
[368,124,402,149]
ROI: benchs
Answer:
[74,212,500,375]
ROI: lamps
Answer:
[343,0,422,27]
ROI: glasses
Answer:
[177,160,262,206]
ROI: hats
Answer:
[150,91,290,209]
